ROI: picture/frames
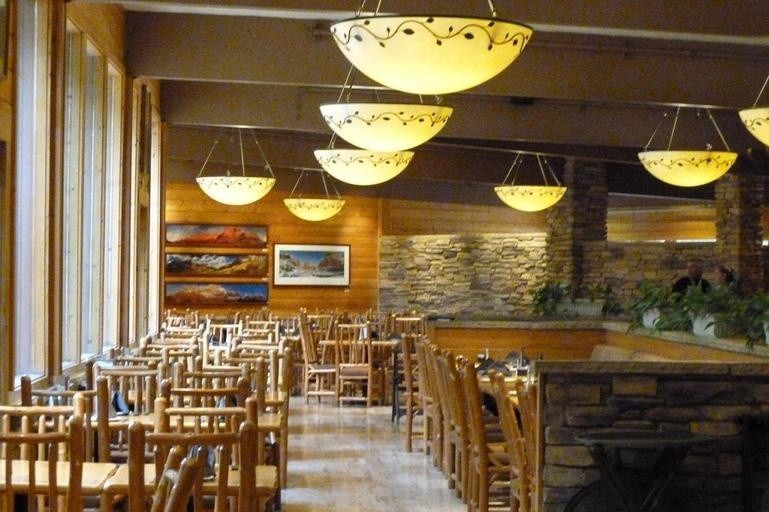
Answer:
[165,223,269,248]
[163,280,269,305]
[164,253,269,278]
[272,242,351,289]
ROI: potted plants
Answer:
[527,273,769,346]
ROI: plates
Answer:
[474,354,531,396]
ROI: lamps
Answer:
[284,172,347,224]
[196,128,277,206]
[637,107,739,188]
[319,64,454,152]
[313,130,415,187]
[738,75,769,147]
[493,154,567,211]
[328,0,535,96]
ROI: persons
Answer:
[710,263,736,293]
[669,258,711,304]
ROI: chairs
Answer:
[588,343,637,362]
[630,352,669,362]
